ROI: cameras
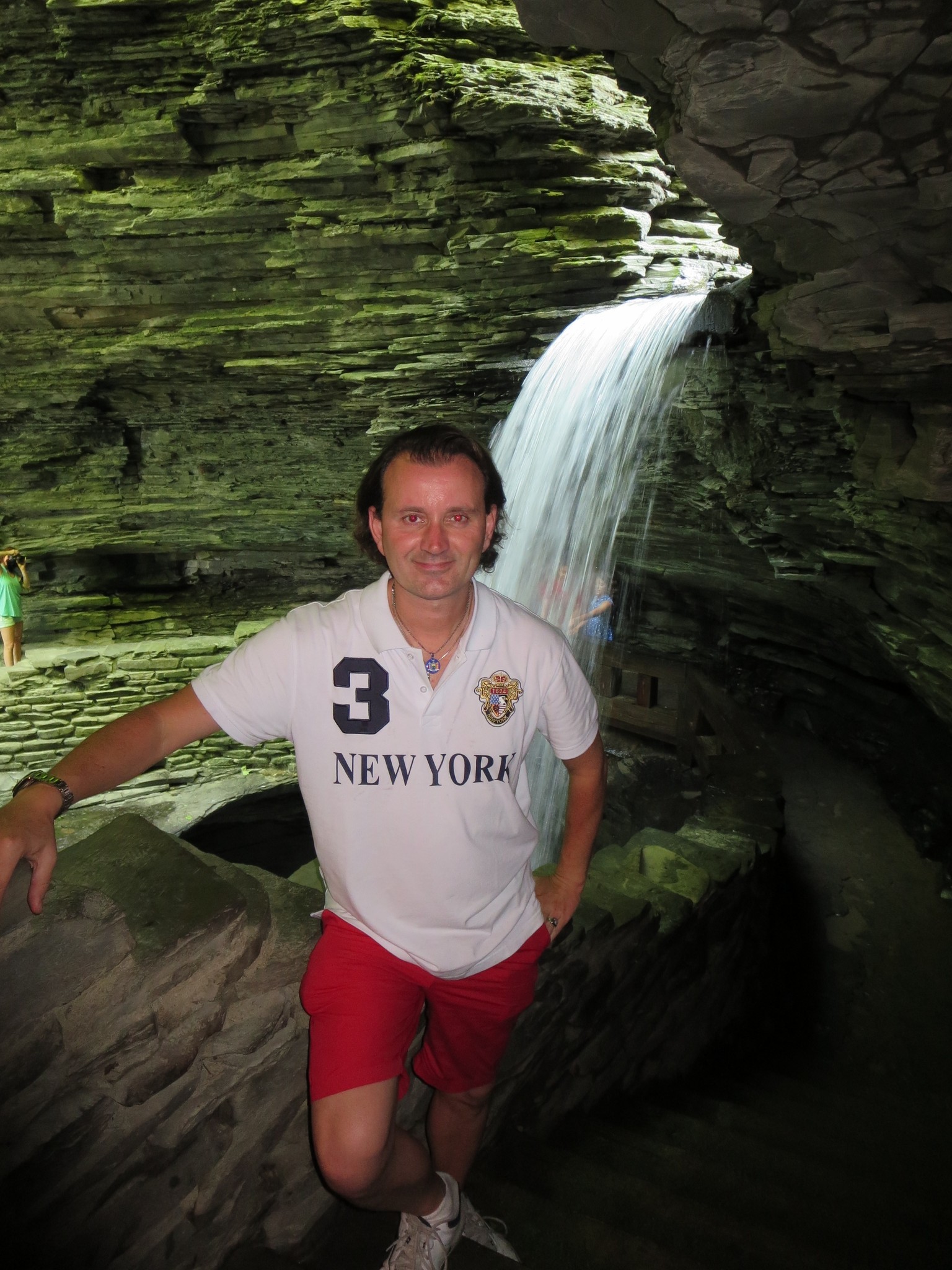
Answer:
[10,553,25,566]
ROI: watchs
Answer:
[12,770,72,819]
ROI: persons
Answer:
[0,546,30,667]
[0,420,618,1270]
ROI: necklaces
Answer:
[392,581,474,679]
[392,585,470,674]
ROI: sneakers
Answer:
[461,1196,521,1263]
[380,1173,467,1270]
